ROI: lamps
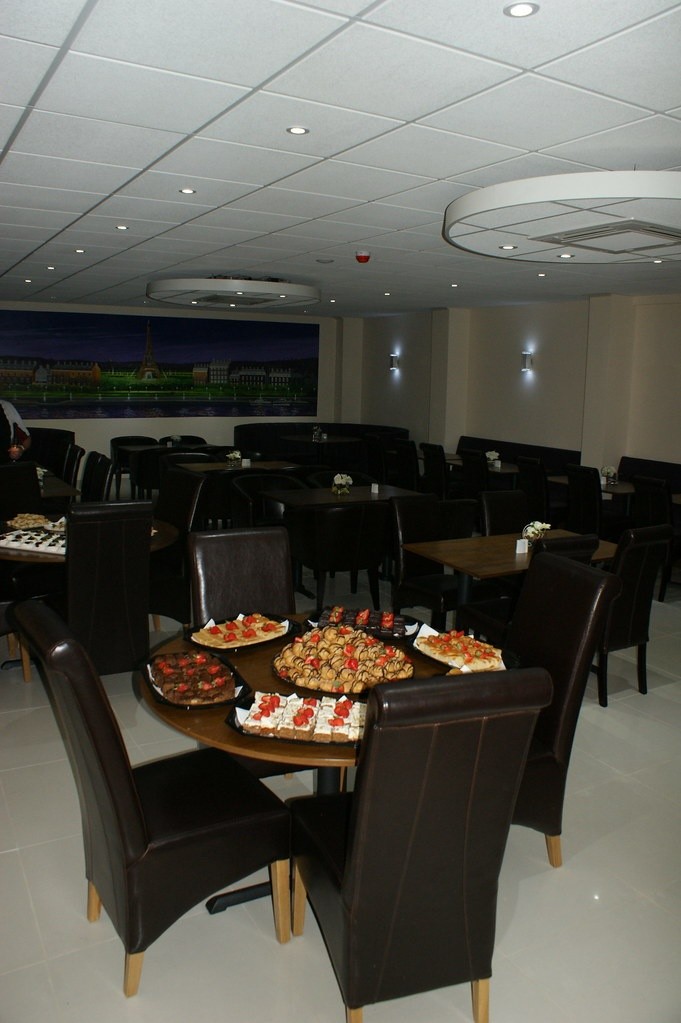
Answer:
[389,353,400,372]
[520,351,533,374]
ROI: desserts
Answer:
[150,606,503,743]
[0,513,66,555]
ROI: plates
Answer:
[225,694,367,746]
[412,624,507,670]
[271,647,416,698]
[302,608,416,640]
[6,519,52,531]
[43,525,66,536]
[184,611,302,652]
[142,652,252,709]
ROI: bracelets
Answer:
[18,445,25,451]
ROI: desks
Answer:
[594,471,640,514]
[172,457,307,527]
[280,433,361,465]
[130,625,500,915]
[260,480,424,593]
[35,464,81,509]
[402,527,617,613]
[115,441,209,498]
[0,513,180,670]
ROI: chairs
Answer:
[0,421,681,1023]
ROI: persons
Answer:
[0,399,32,465]
